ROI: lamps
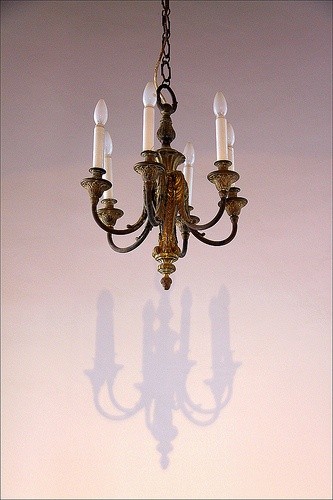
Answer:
[79,0,249,292]
